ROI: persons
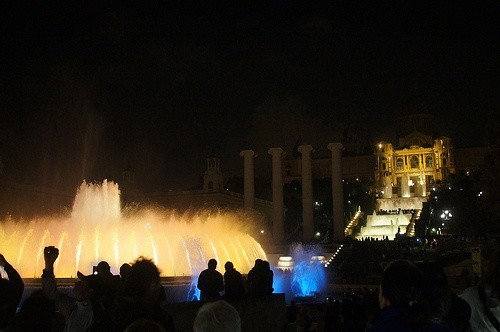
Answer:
[197,259,224,303]
[247,259,274,295]
[42,245,103,332]
[223,261,243,300]
[90,256,176,332]
[344,248,500,332]
[0,254,65,332]
[193,301,241,332]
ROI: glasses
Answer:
[75,282,85,289]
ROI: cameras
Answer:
[45,247,56,255]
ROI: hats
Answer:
[77,271,105,299]
